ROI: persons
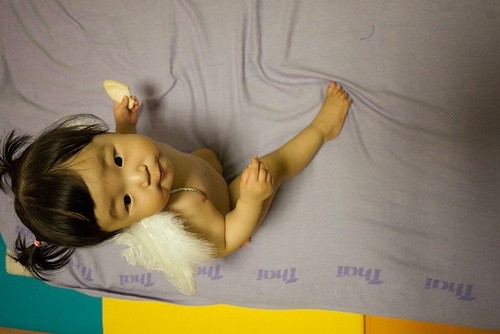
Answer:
[0,77,354,297]
